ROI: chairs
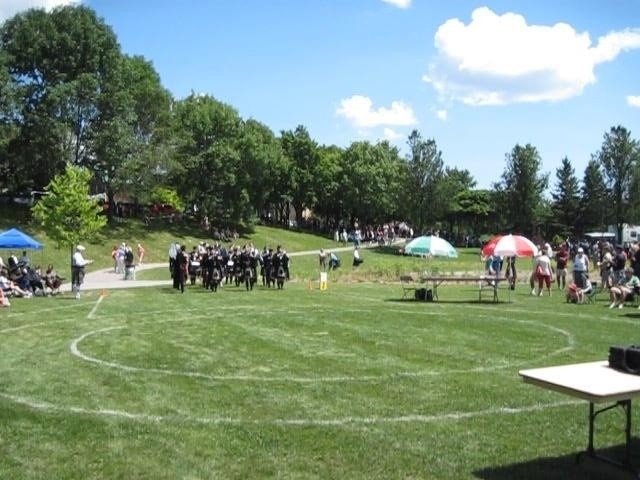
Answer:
[579,281,598,304]
[1,259,61,298]
[611,283,637,308]
[400,273,418,299]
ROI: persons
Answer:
[169,241,292,291]
[112,241,144,281]
[72,245,93,300]
[399,219,482,248]
[486,253,516,290]
[0,251,65,307]
[320,246,340,269]
[201,215,241,242]
[96,197,201,226]
[352,248,363,267]
[528,237,640,309]
[259,213,399,247]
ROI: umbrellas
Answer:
[479,233,539,304]
[403,229,459,301]
[0,226,43,273]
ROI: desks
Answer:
[517,356,639,479]
[422,277,512,302]
